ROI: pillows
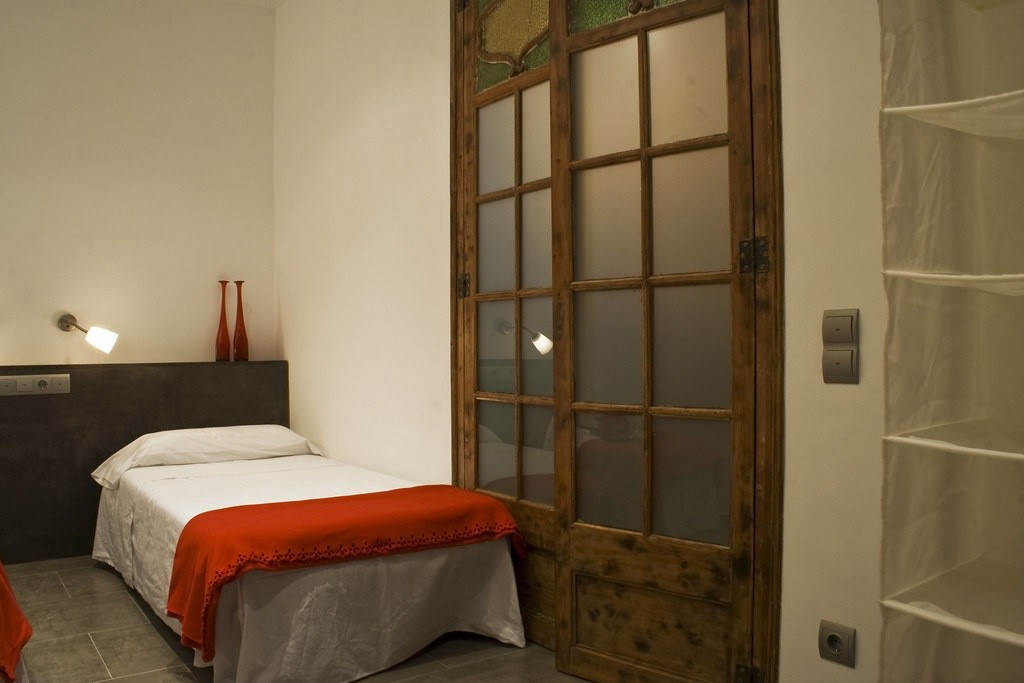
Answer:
[478,423,502,442]
[90,425,324,490]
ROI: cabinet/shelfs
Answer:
[875,0,1024,683]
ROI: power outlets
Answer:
[0,373,70,396]
[818,619,856,668]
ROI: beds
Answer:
[477,411,723,526]
[0,361,529,683]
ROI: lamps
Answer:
[498,320,554,355]
[57,313,119,354]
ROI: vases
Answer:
[215,280,230,361]
[233,281,249,362]
